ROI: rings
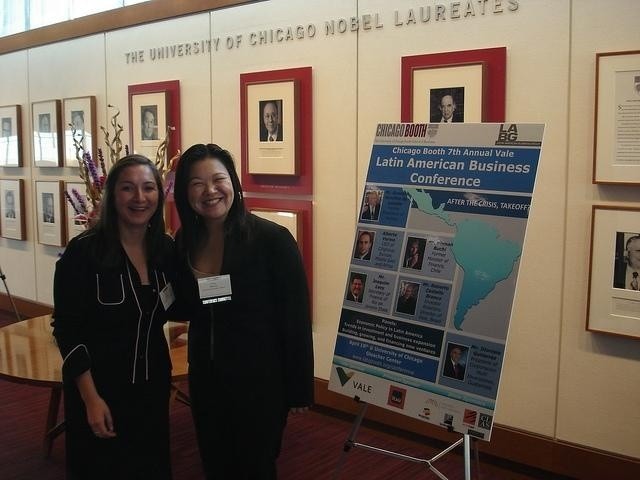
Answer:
[95,431,101,435]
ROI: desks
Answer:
[1,312,191,458]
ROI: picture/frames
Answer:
[35,179,66,248]
[238,66,314,197]
[399,47,508,122]
[584,204,640,340]
[65,182,96,241]
[0,178,27,242]
[164,194,181,234]
[63,96,99,169]
[0,104,23,169]
[127,80,182,168]
[31,100,61,168]
[241,196,316,327]
[592,50,640,187]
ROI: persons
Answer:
[173,142,316,480]
[362,191,381,220]
[71,112,85,141]
[42,194,54,222]
[354,231,373,261]
[2,118,11,137]
[613,236,640,291]
[50,153,176,480]
[5,191,15,218]
[261,102,282,141]
[403,237,422,270]
[141,108,157,140]
[39,114,50,133]
[443,347,464,380]
[430,95,463,122]
[346,274,364,303]
[396,281,417,315]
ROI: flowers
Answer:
[66,110,178,234]
